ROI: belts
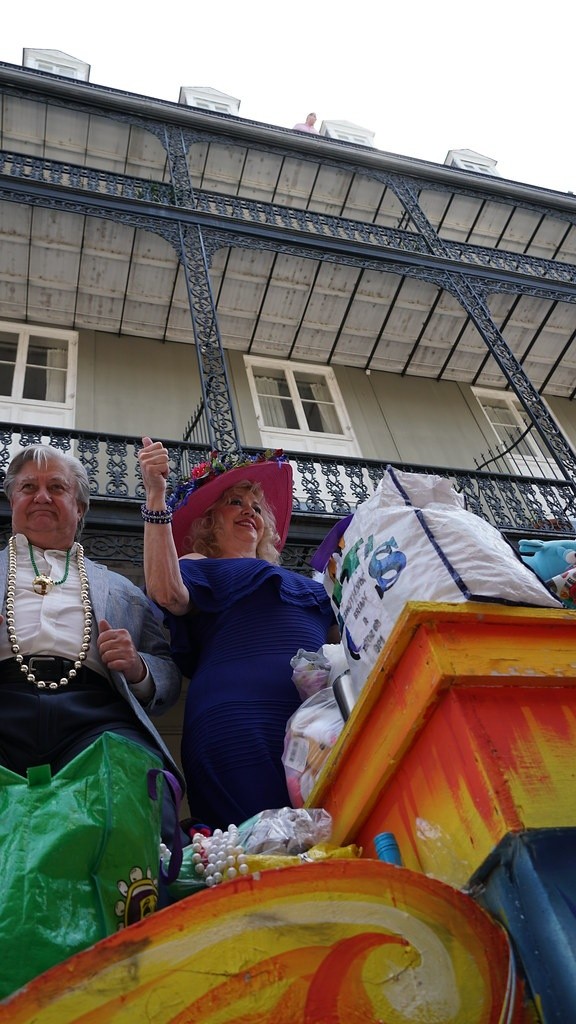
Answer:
[0,654,110,693]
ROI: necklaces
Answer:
[28,542,70,594]
[193,824,248,884]
[6,535,92,690]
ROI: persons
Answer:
[293,113,319,134]
[138,437,341,834]
[0,443,190,851]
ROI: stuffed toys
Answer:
[518,539,576,580]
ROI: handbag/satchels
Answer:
[309,464,568,692]
[1,731,183,1004]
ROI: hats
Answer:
[166,443,293,558]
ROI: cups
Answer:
[332,669,357,722]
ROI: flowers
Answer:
[165,447,290,512]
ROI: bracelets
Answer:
[141,504,172,524]
